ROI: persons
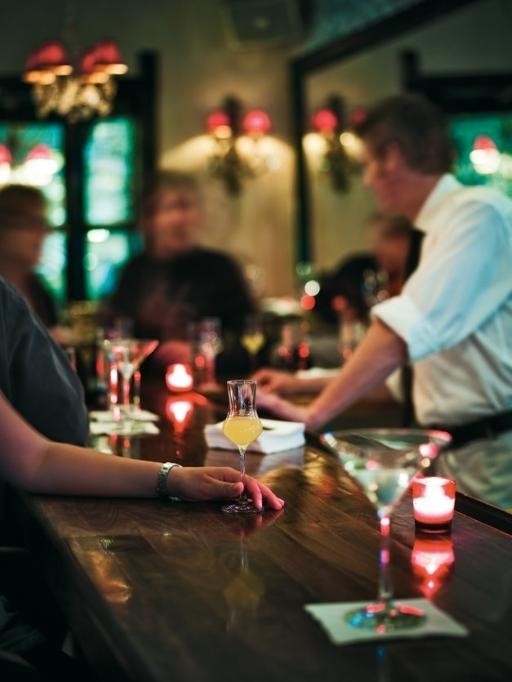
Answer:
[1,396,287,517]
[97,167,252,386]
[1,278,90,450]
[0,185,59,327]
[254,95,511,513]
[255,214,415,426]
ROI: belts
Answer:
[429,407,512,450]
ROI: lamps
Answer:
[199,92,273,202]
[303,88,378,201]
[11,0,131,133]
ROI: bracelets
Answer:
[158,459,184,501]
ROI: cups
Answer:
[410,478,455,538]
[165,363,195,394]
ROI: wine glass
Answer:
[96,338,160,434]
[317,426,454,630]
[222,379,264,517]
